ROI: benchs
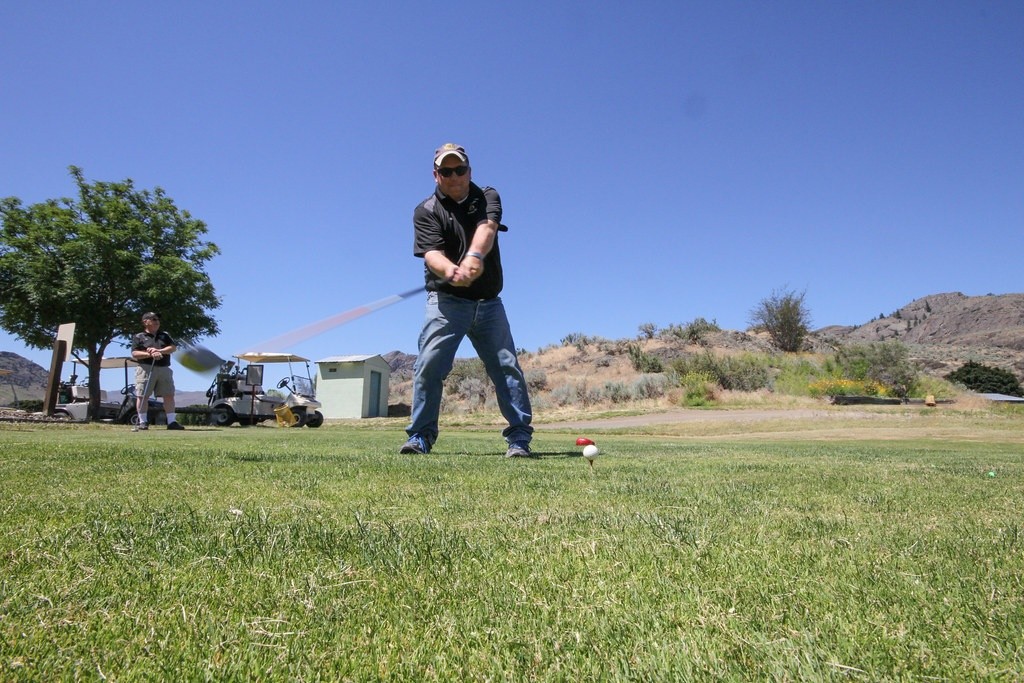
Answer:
[238,380,283,403]
[72,385,122,408]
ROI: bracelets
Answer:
[160,349,164,354]
[466,251,484,260]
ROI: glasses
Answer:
[152,317,161,322]
[437,166,469,177]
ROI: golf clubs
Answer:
[243,276,451,362]
[131,357,157,432]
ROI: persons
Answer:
[400,144,535,457]
[131,312,185,431]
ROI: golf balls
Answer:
[582,445,599,459]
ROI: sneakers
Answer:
[400,433,432,454]
[506,441,532,457]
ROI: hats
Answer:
[142,311,161,319]
[433,143,468,166]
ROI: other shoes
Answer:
[168,421,184,430]
[139,421,148,429]
